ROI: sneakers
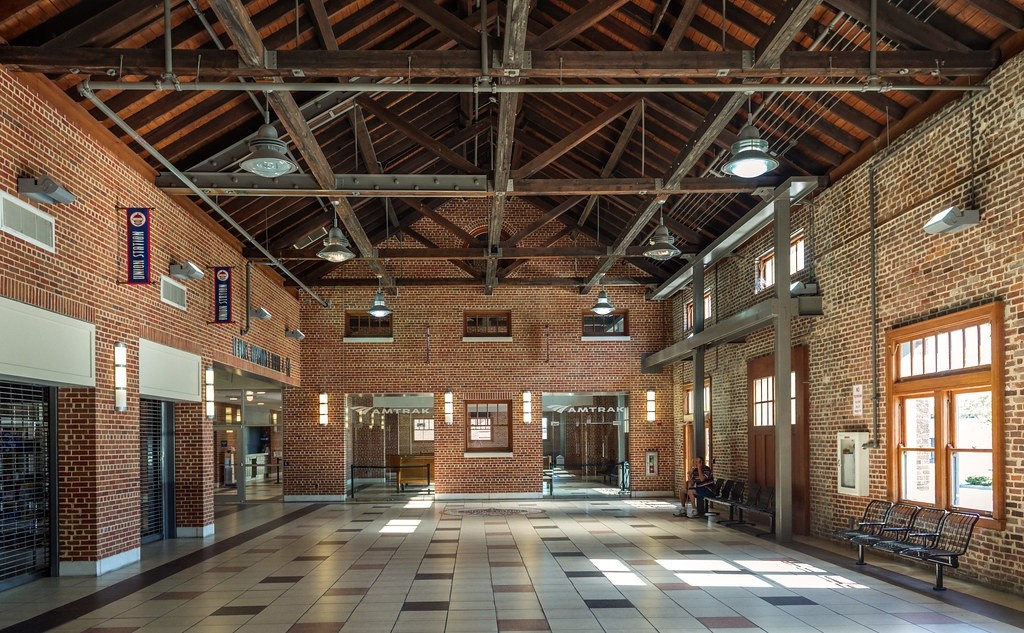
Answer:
[687,510,700,519]
[673,509,687,517]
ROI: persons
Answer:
[672,457,714,519]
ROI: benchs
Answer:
[836,498,981,592]
[394,452,435,491]
[595,460,618,486]
[702,478,775,535]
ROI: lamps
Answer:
[444,387,454,426]
[236,88,299,179]
[286,329,306,341]
[316,197,355,263]
[16,172,78,206]
[719,91,780,178]
[113,342,128,412]
[364,273,395,319]
[205,367,216,419]
[249,307,272,321]
[646,389,657,423]
[169,261,205,281]
[521,390,533,425]
[589,272,615,315]
[318,391,330,427]
[922,206,981,236]
[642,198,682,261]
